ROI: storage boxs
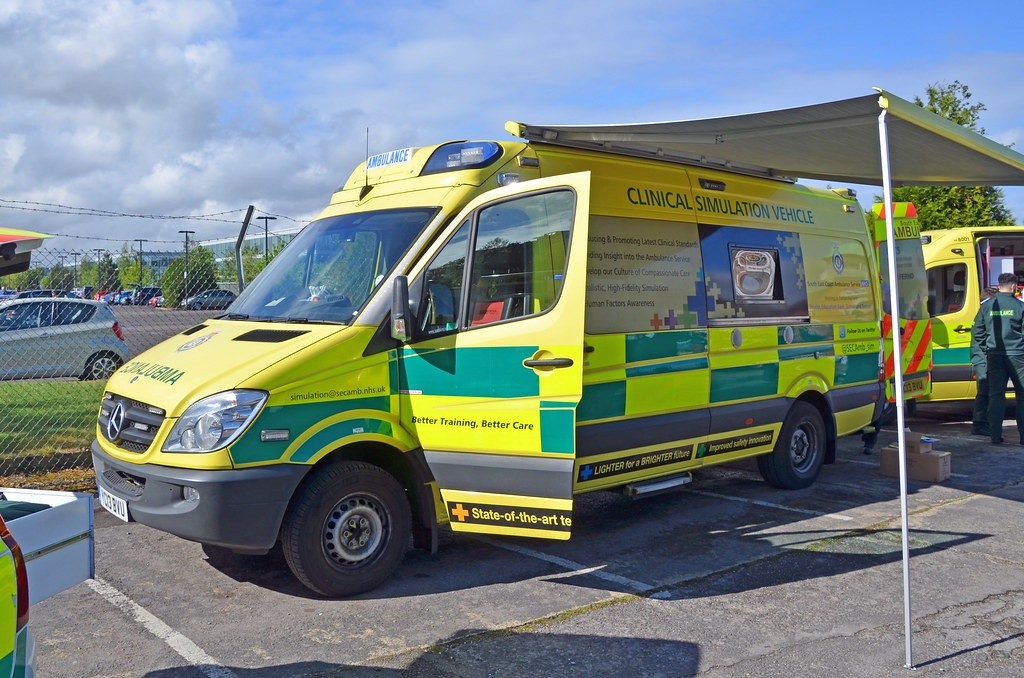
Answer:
[905,427,932,454]
[879,446,951,482]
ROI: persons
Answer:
[126,294,132,306]
[861,401,911,454]
[136,290,141,306]
[970,273,1024,445]
[112,289,121,307]
[51,290,58,298]
[1,286,9,295]
[15,287,21,293]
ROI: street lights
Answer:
[256,216,277,265]
[93,248,105,300]
[71,253,81,288]
[133,239,148,306]
[33,261,41,285]
[177,230,195,306]
[58,255,68,298]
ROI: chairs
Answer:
[940,271,965,313]
[408,269,429,327]
[927,277,936,315]
[467,235,522,326]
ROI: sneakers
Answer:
[972,425,992,436]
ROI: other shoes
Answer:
[1020,440,1024,445]
[863,441,875,454]
[991,438,1004,444]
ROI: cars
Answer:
[0,297,130,380]
[12,290,83,299]
[183,288,238,311]
[93,287,165,307]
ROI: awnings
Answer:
[506,91,1024,669]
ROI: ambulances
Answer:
[864,201,933,416]
[905,225,1024,403]
[89,139,886,597]
